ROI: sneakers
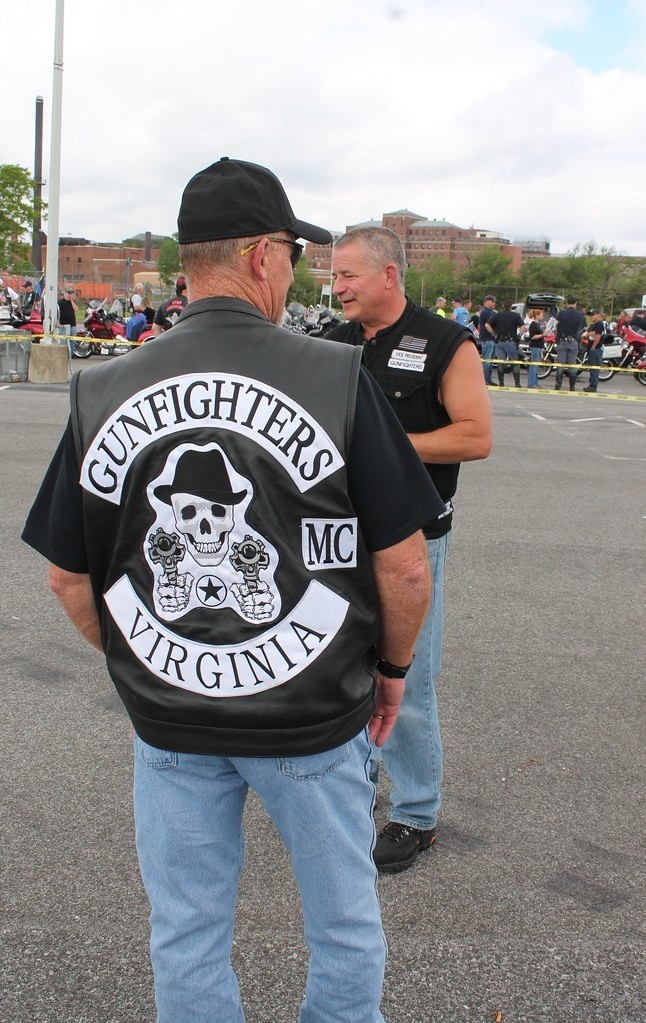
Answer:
[372,820,438,874]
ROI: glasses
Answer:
[240,236,304,269]
[589,313,598,316]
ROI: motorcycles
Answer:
[286,302,347,337]
[70,297,166,359]
[0,304,43,343]
[501,323,646,386]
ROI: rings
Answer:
[374,715,382,719]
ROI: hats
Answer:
[452,299,461,304]
[22,281,32,287]
[65,288,75,294]
[484,295,498,302]
[177,157,334,245]
[567,298,576,304]
[586,309,599,316]
[176,277,188,290]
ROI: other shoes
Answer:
[491,382,498,387]
[583,385,596,392]
[531,384,545,389]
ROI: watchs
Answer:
[373,654,415,679]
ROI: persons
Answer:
[128,283,155,330]
[55,286,78,358]
[152,277,188,337]
[429,295,603,392]
[20,156,435,1023]
[309,226,492,873]
[0,279,40,328]
[603,310,646,335]
